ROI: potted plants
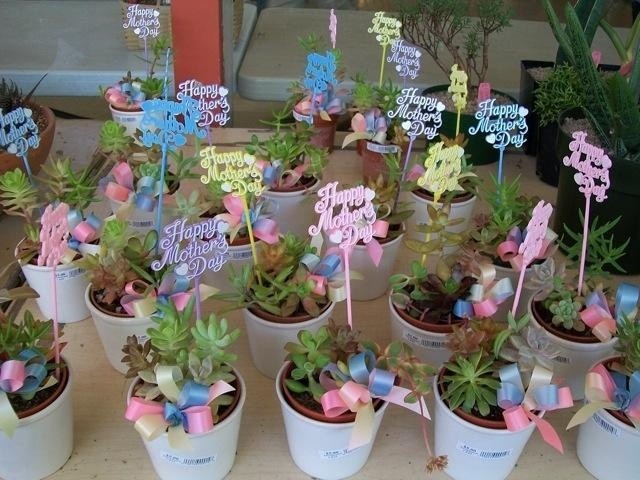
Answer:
[0,0,640,478]
